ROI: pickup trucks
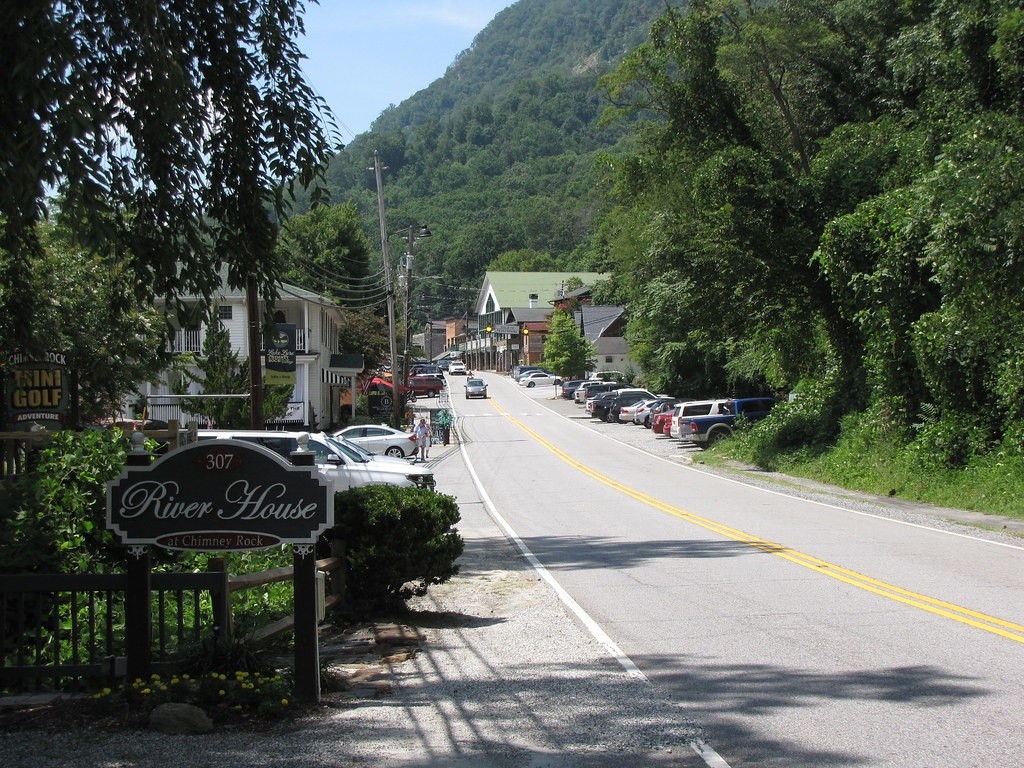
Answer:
[676,397,779,449]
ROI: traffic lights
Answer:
[487,327,491,332]
[524,330,528,334]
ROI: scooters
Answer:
[405,385,417,402]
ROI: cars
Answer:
[449,361,467,375]
[515,366,553,378]
[308,433,414,465]
[519,373,562,388]
[408,364,447,387]
[437,360,451,372]
[515,370,555,382]
[195,429,437,493]
[327,425,419,459]
[102,419,170,454]
[399,377,444,398]
[561,371,736,438]
[464,380,488,399]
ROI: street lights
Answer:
[403,224,433,404]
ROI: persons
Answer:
[723,398,731,415]
[414,419,431,461]
[425,424,433,458]
